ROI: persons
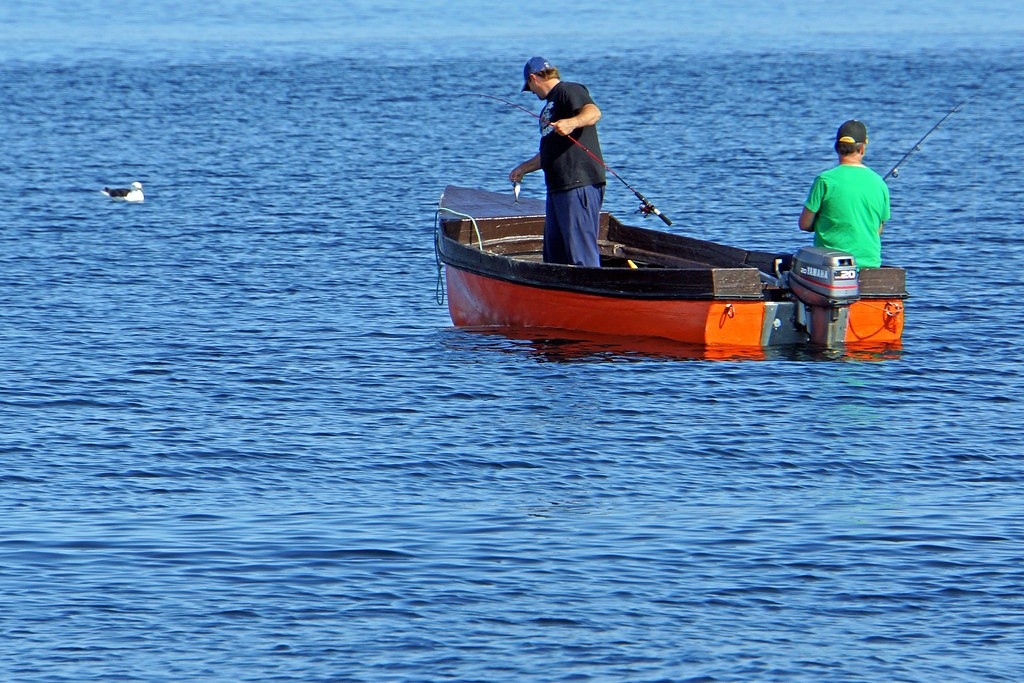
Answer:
[509,56,606,267]
[772,119,891,278]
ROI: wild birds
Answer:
[100,181,144,203]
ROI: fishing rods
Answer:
[881,89,983,182]
[457,92,674,226]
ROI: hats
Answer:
[521,56,552,92]
[836,120,868,144]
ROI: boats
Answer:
[432,185,910,361]
